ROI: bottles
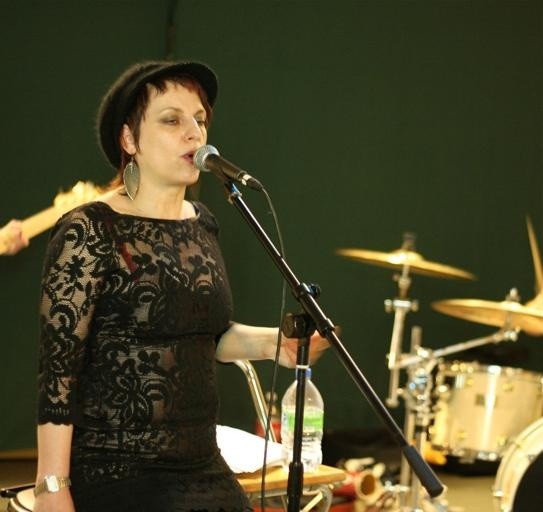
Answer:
[279,363,326,473]
[257,390,281,446]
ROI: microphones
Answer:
[192,145,264,190]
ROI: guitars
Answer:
[0,181,125,253]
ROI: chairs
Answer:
[215,356,346,511]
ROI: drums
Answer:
[491,417,543,512]
[431,361,543,464]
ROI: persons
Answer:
[32,62,341,511]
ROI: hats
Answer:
[98,62,219,171]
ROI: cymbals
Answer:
[334,249,474,281]
[433,299,543,335]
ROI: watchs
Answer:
[33,475,72,496]
